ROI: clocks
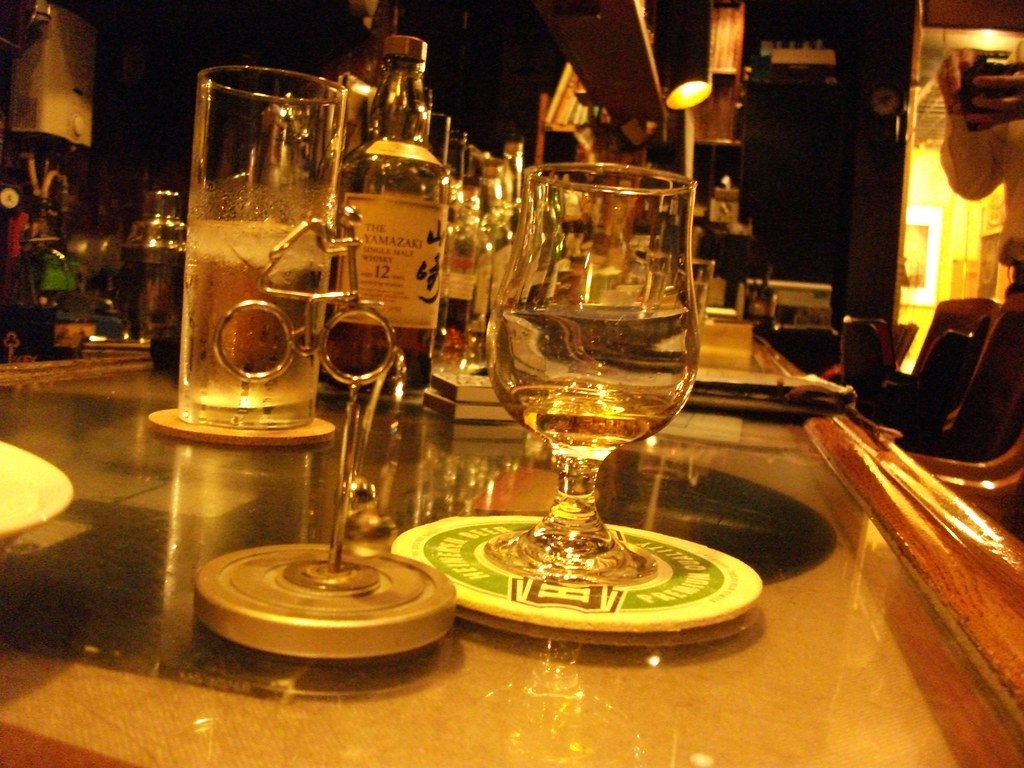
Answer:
[866,82,906,152]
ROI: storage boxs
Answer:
[737,278,834,329]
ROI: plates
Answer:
[0,441,75,559]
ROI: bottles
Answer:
[332,33,562,391]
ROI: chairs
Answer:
[819,295,1022,535]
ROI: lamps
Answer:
[663,51,713,110]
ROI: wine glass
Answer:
[487,164,702,587]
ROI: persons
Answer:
[938,34,1024,294]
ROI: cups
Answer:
[178,66,348,429]
